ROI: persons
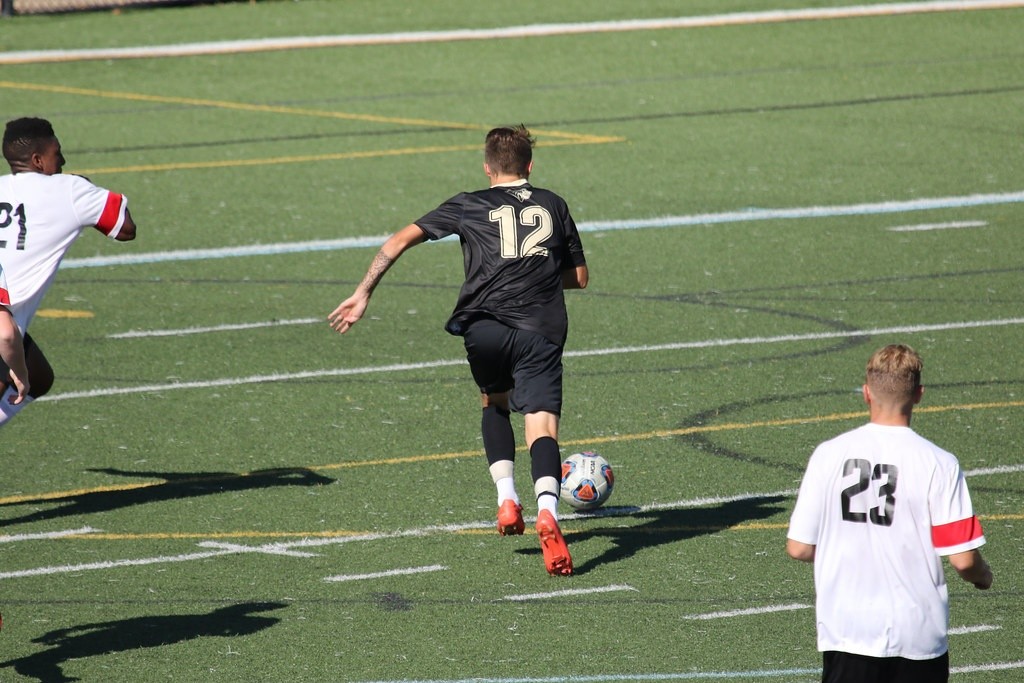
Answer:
[786,345,993,683]
[1,118,135,426]
[326,126,589,577]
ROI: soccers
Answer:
[559,452,615,510]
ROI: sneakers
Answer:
[534,509,573,575]
[495,500,526,535]
